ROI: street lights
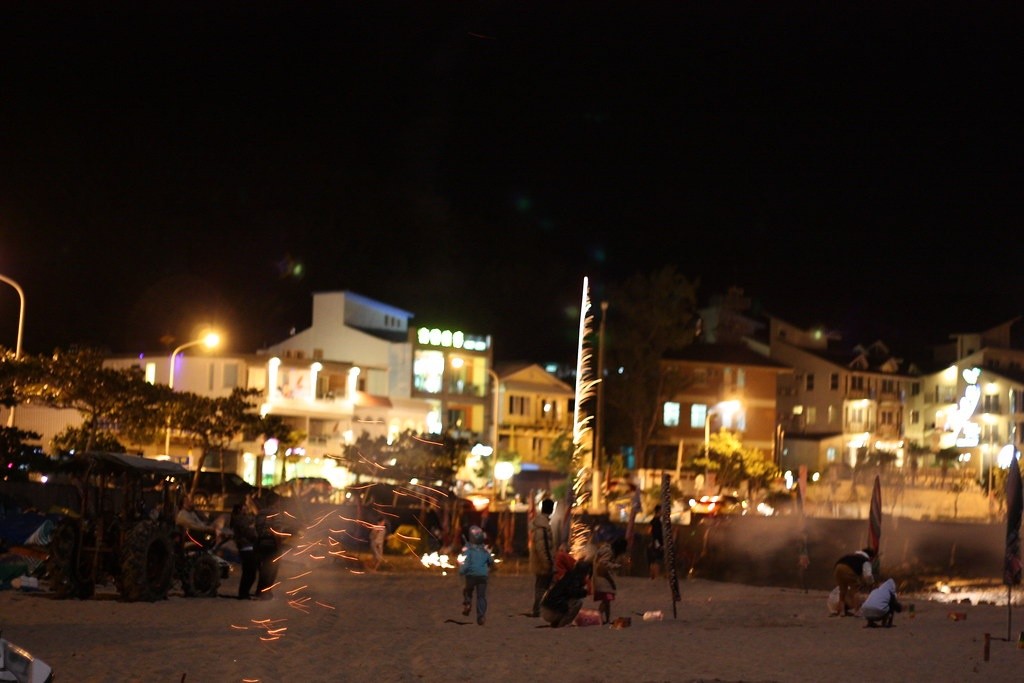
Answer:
[165,332,218,460]
[452,358,499,508]
[705,399,738,492]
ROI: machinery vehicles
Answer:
[47,452,222,604]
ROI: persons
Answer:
[459,526,494,626]
[828,584,863,617]
[836,548,876,618]
[230,502,258,602]
[592,538,628,625]
[544,548,584,628]
[254,493,285,599]
[649,505,665,583]
[530,499,555,618]
[859,578,902,628]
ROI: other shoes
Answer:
[839,611,846,617]
[882,622,898,627]
[477,615,485,625]
[462,605,471,616]
[847,609,863,617]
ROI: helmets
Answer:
[468,525,484,544]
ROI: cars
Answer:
[691,493,740,517]
